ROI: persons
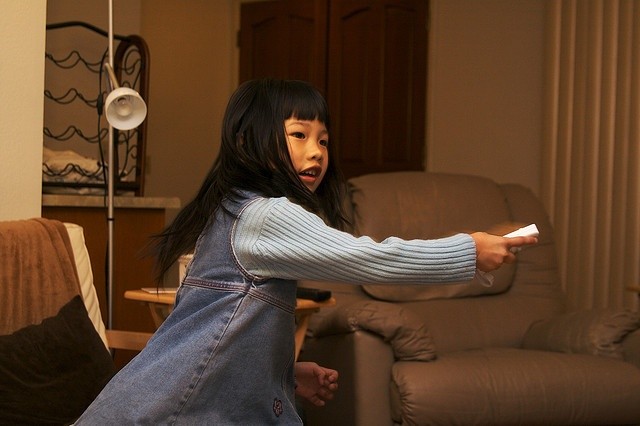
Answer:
[71,77,538,426]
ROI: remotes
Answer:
[295,287,332,302]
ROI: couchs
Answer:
[0,217,156,425]
[312,169,639,426]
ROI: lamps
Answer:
[103,62,150,330]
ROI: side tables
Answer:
[121,286,336,362]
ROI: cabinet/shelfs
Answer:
[45,19,183,210]
